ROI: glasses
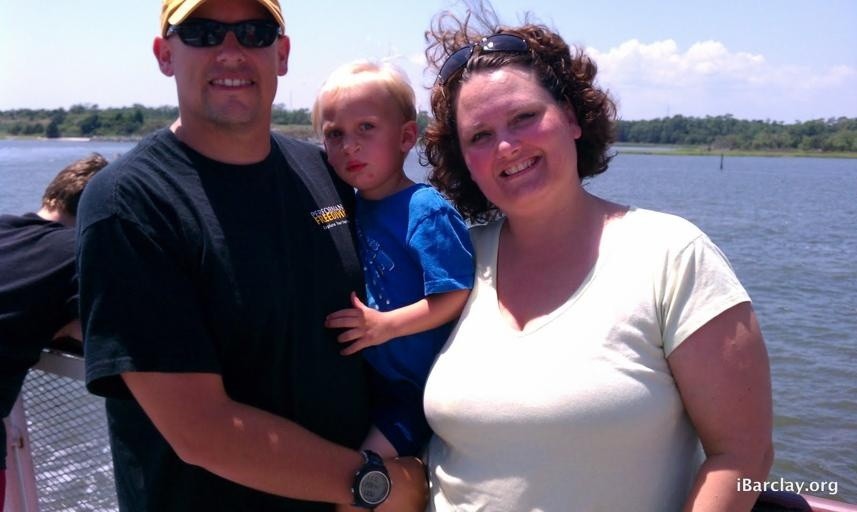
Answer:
[166,17,282,48]
[437,34,529,87]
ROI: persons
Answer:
[0,152,114,511]
[74,0,431,512]
[417,1,773,511]
[307,61,476,465]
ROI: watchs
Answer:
[348,445,392,507]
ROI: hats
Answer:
[159,0,285,39]
